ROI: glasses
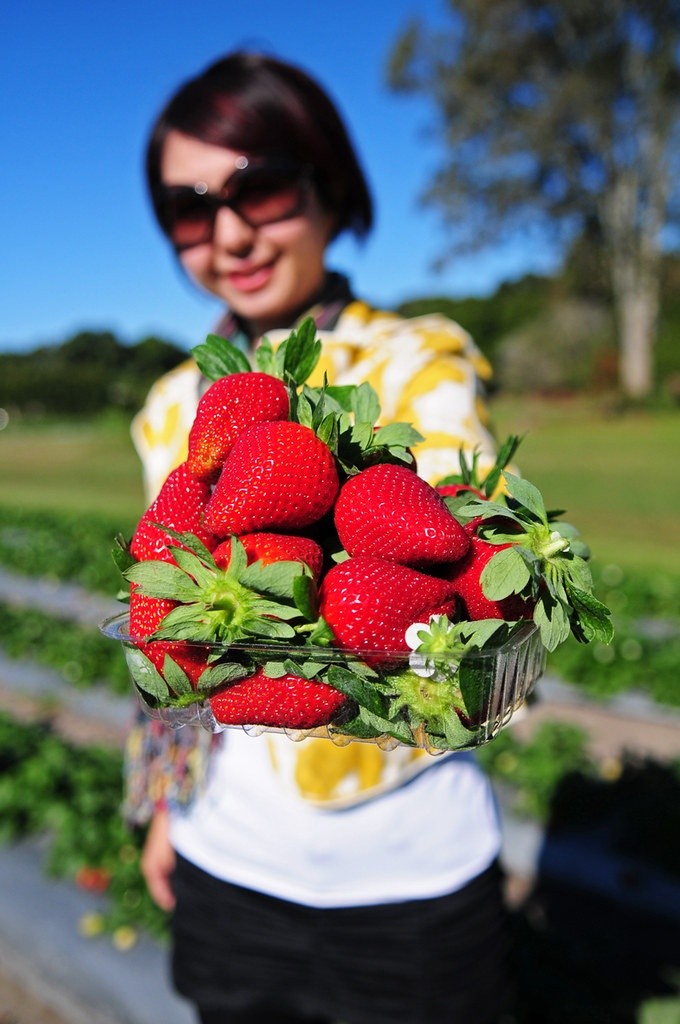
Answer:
[153,157,322,250]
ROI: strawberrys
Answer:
[111,315,615,752]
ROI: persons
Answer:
[131,52,524,1024]
